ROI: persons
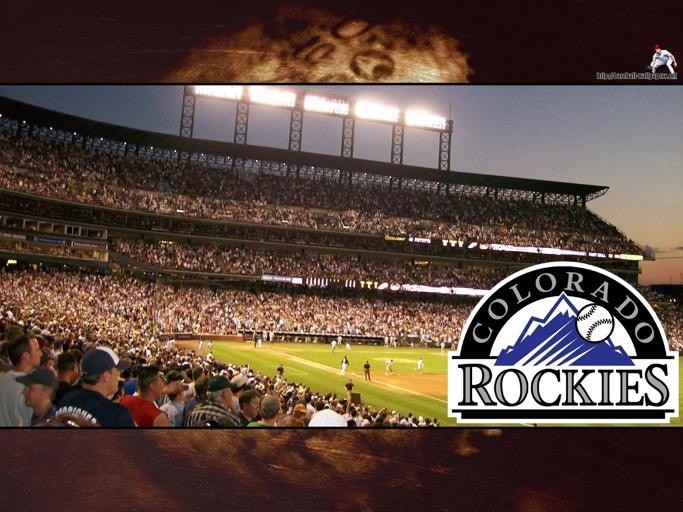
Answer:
[446,192,683,351]
[6,136,446,428]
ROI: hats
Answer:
[166,373,248,394]
[81,346,129,377]
[261,397,283,411]
[15,367,60,388]
